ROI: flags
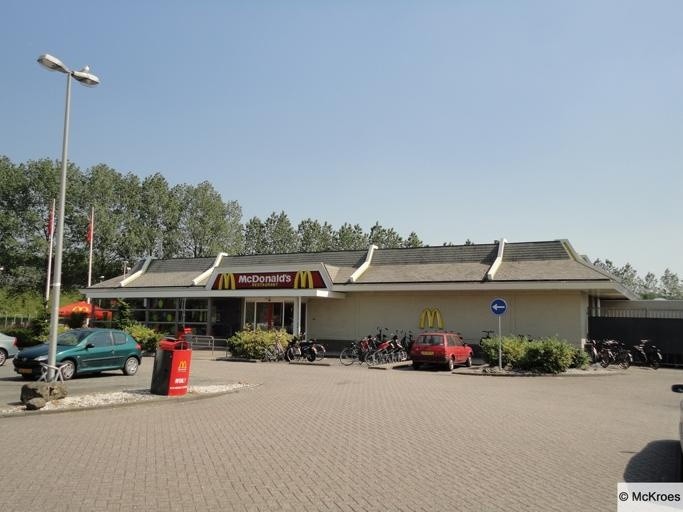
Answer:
[46,207,52,239]
[86,224,90,241]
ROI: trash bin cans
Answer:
[150,340,191,395]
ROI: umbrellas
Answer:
[47,300,111,319]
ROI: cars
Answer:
[13,328,142,380]
[0,332,20,366]
[411,333,473,370]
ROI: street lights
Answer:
[36,54,98,383]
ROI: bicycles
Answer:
[287,330,325,362]
[341,328,413,365]
[586,339,663,369]
